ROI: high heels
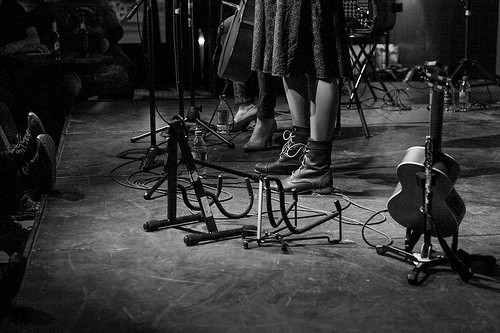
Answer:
[243,119,277,151]
[229,111,259,131]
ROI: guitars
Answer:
[386,59,468,246]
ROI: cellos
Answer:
[192,0,255,84]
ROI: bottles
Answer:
[459,76,471,111]
[444,78,455,112]
[77,20,89,59]
[192,131,207,178]
[218,95,229,134]
[49,19,61,60]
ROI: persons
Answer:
[0,0,144,321]
[216,14,283,151]
[250,0,355,191]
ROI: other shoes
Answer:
[20,134,56,193]
[9,193,40,220]
[5,112,45,167]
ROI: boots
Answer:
[264,138,333,194]
[254,126,310,175]
